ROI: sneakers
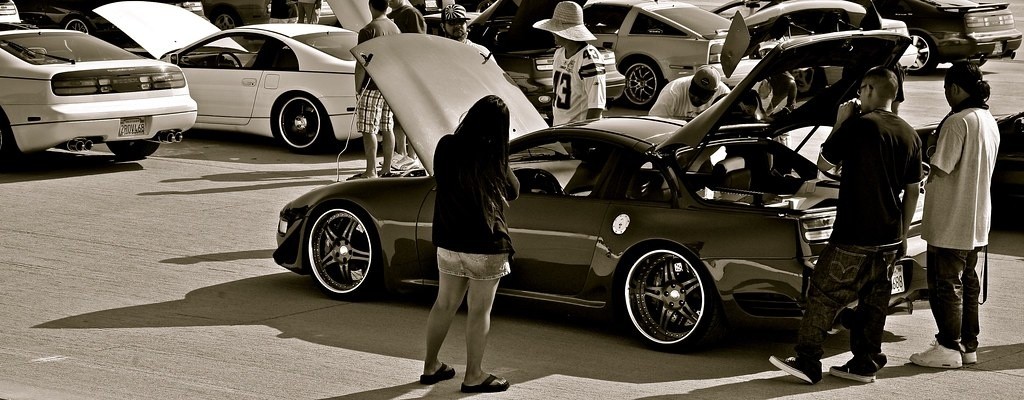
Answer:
[829,359,877,382]
[392,155,420,170]
[909,340,963,368]
[768,355,822,383]
[379,152,403,167]
[961,351,977,364]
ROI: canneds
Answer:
[853,99,861,112]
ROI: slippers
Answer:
[461,374,510,393]
[420,362,456,384]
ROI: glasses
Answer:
[857,84,873,96]
[446,19,466,26]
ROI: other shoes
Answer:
[377,170,390,178]
[345,173,368,180]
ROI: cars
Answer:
[82,0,375,153]
[418,0,911,122]
[873,0,1022,76]
[273,28,949,354]
[0,21,198,177]
[912,111,1024,228]
[0,0,120,39]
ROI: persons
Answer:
[268,0,323,24]
[345,0,403,180]
[747,33,797,156]
[647,65,737,174]
[769,66,926,386]
[440,4,498,73]
[379,0,427,171]
[909,61,1001,370]
[420,95,521,393]
[531,1,607,155]
[888,60,905,115]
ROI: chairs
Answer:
[713,156,751,187]
[561,145,614,198]
[277,49,298,69]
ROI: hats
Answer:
[533,1,598,41]
[689,65,721,100]
[440,4,471,20]
